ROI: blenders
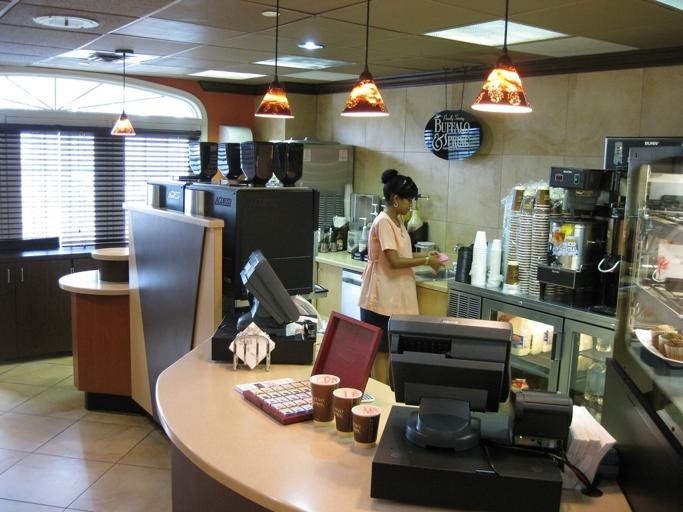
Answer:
[347,194,379,252]
[536,166,615,306]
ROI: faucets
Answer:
[455,243,463,253]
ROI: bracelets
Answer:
[358,168,447,385]
[424,257,430,265]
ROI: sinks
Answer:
[415,269,457,282]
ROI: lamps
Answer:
[110,47,136,137]
[253,1,295,119]
[340,1,389,119]
[467,0,532,113]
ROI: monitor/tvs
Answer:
[236,250,300,337]
[385,312,513,451]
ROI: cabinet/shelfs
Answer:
[479,296,617,424]
[0,257,71,367]
[311,251,449,383]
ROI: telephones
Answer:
[292,295,323,330]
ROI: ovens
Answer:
[341,268,362,321]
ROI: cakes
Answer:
[658,333,683,354]
[652,325,678,351]
[664,338,683,361]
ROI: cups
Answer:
[331,387,363,432]
[471,230,487,288]
[351,404,381,449]
[488,239,501,285]
[536,189,550,262]
[502,185,535,297]
[551,189,571,216]
[309,374,339,423]
